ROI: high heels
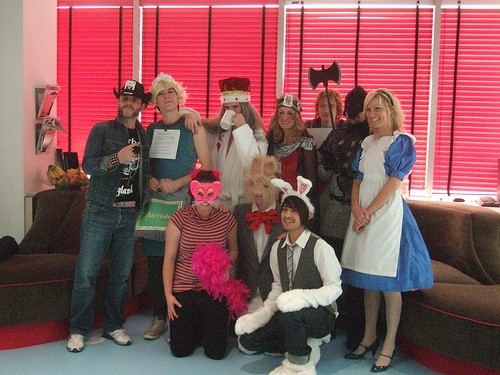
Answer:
[345,338,380,360]
[371,346,396,372]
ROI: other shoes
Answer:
[268,359,318,375]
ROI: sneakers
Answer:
[143,319,168,339]
[102,328,131,346]
[66,334,85,352]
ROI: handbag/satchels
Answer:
[133,191,191,241]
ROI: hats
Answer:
[113,79,152,105]
[218,75,250,104]
[273,95,302,114]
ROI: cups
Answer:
[220,110,235,130]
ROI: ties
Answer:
[286,247,294,291]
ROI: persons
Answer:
[216,154,288,354]
[181,77,367,351]
[233,177,344,375]
[63,70,212,359]
[340,89,433,372]
[162,171,239,360]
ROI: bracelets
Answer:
[110,153,120,166]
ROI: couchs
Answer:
[402,201,500,375]
[0,189,147,351]
